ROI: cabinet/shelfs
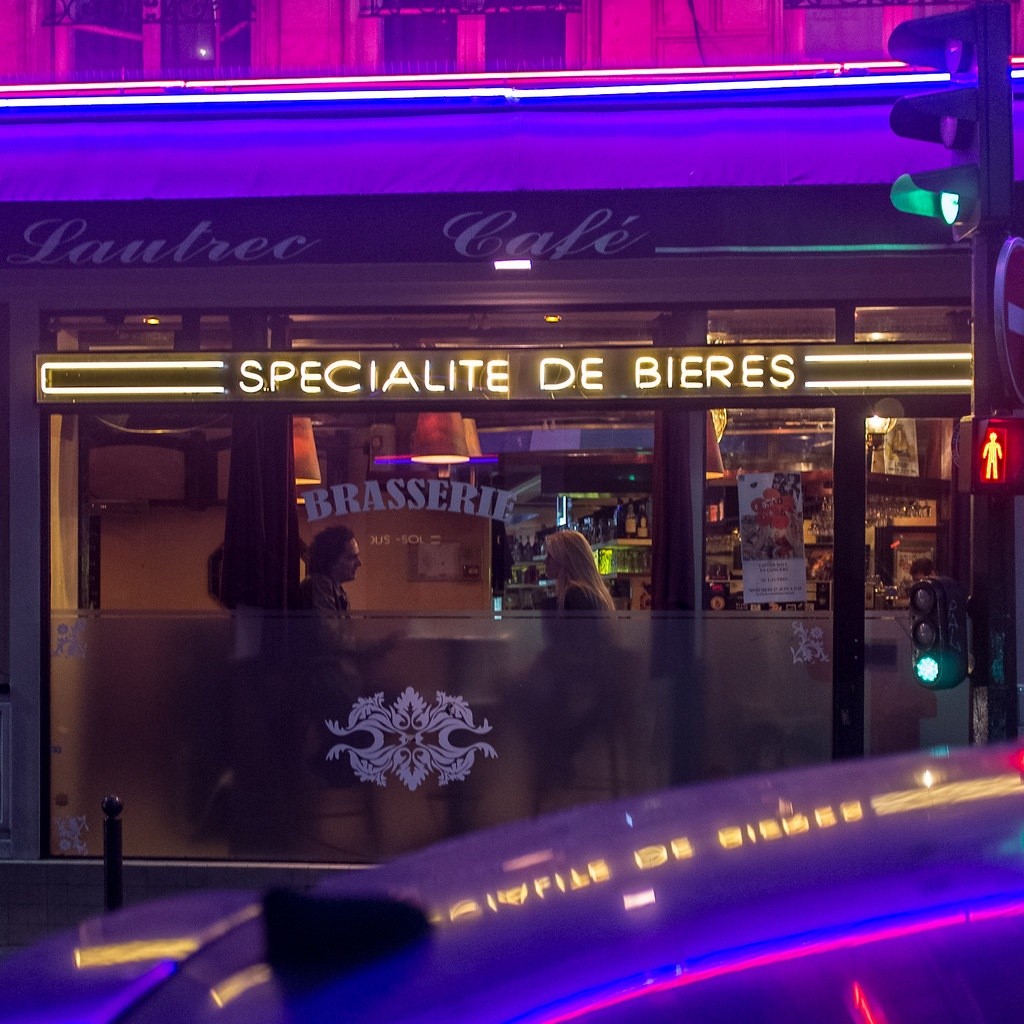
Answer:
[501,496,654,620]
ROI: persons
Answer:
[501,531,620,785]
[293,524,409,788]
[906,556,937,584]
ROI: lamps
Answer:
[865,417,897,452]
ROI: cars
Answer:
[0,746,1024,1024]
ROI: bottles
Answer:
[505,495,650,583]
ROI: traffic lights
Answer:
[958,414,1023,497]
[908,576,967,690]
[888,0,990,242]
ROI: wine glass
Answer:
[806,492,932,536]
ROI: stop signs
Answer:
[994,236,1024,405]
